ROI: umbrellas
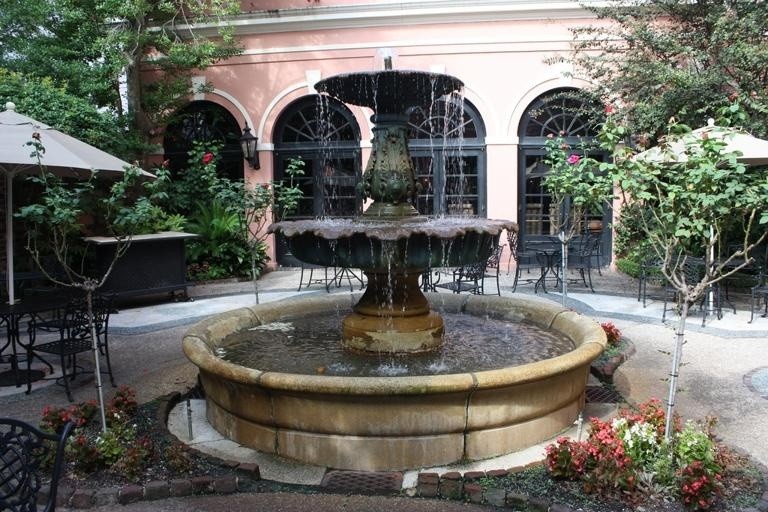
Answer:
[0,102,158,355]
[626,118,768,316]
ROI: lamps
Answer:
[239,119,260,170]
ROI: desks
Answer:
[80,232,202,315]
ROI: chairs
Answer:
[637,228,768,327]
[435,227,604,297]
[0,268,119,512]
